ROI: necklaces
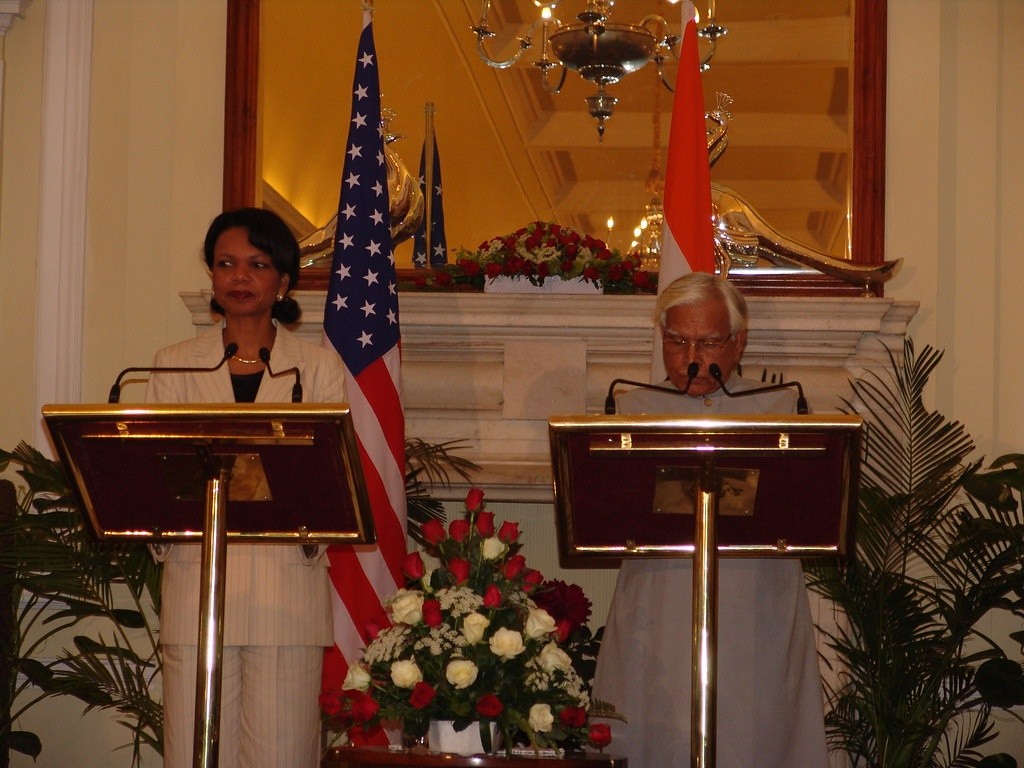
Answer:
[231,355,261,364]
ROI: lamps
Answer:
[468,0,727,143]
[255,1,853,267]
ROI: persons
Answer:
[142,206,349,768]
[583,271,830,768]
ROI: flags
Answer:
[321,9,408,715]
[411,134,449,269]
[649,0,716,384]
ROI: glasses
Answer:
[659,329,733,353]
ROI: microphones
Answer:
[108,343,239,403]
[605,362,699,415]
[259,346,303,403]
[710,362,808,416]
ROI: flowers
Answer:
[319,487,628,756]
[415,220,655,291]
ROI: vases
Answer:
[425,716,502,755]
